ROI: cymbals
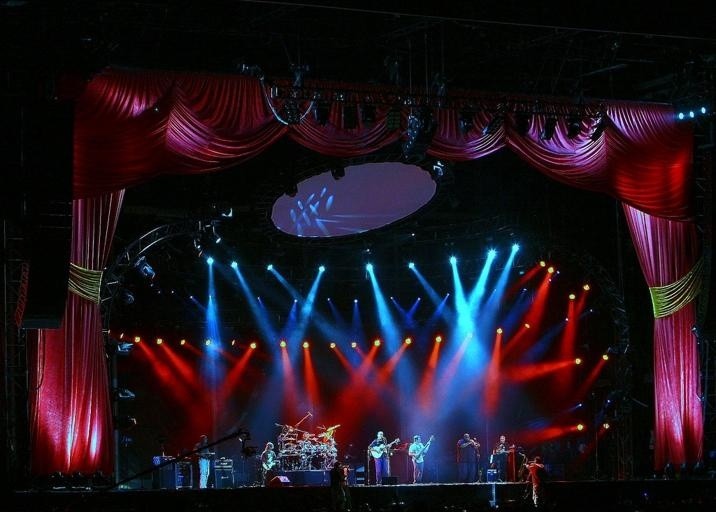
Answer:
[327,424,340,431]
[318,433,328,437]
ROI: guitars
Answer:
[371,438,399,459]
[262,457,278,470]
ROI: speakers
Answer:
[269,475,290,487]
[14,99,75,328]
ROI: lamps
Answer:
[264,87,613,142]
[102,216,226,433]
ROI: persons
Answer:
[295,432,311,450]
[408,435,431,484]
[457,434,480,483]
[260,442,277,480]
[493,436,509,483]
[526,456,545,508]
[193,435,210,489]
[368,431,391,485]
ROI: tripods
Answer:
[299,448,335,470]
